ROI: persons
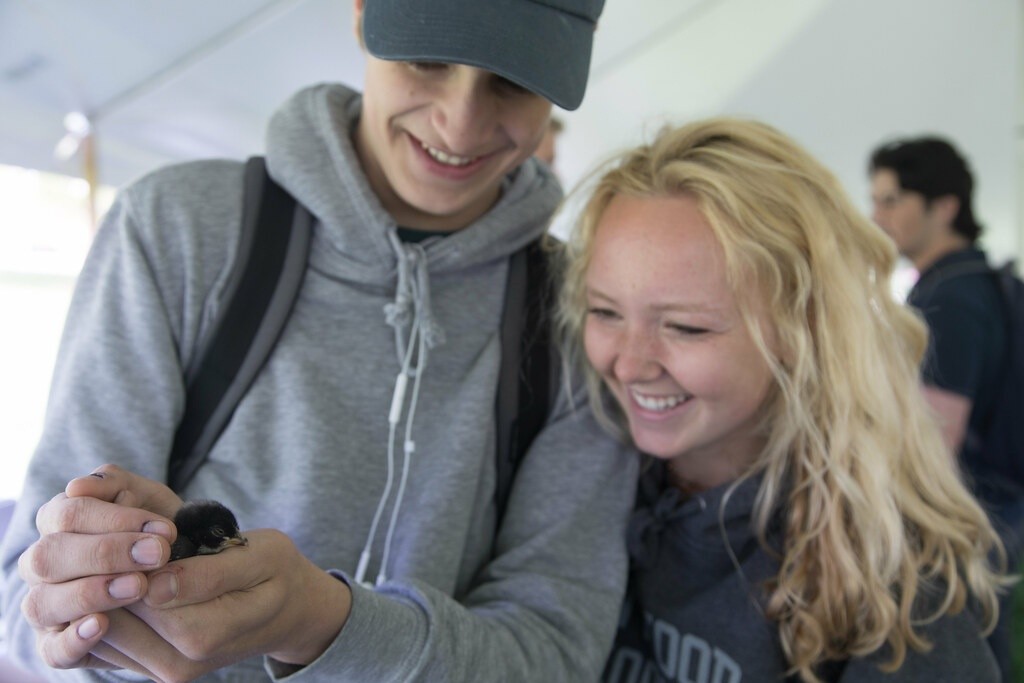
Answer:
[865,135,1024,683]
[554,113,1001,683]
[0,0,637,683]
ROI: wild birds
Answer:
[171,497,249,561]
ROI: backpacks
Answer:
[969,266,1024,510]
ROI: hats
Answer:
[362,0,607,111]
